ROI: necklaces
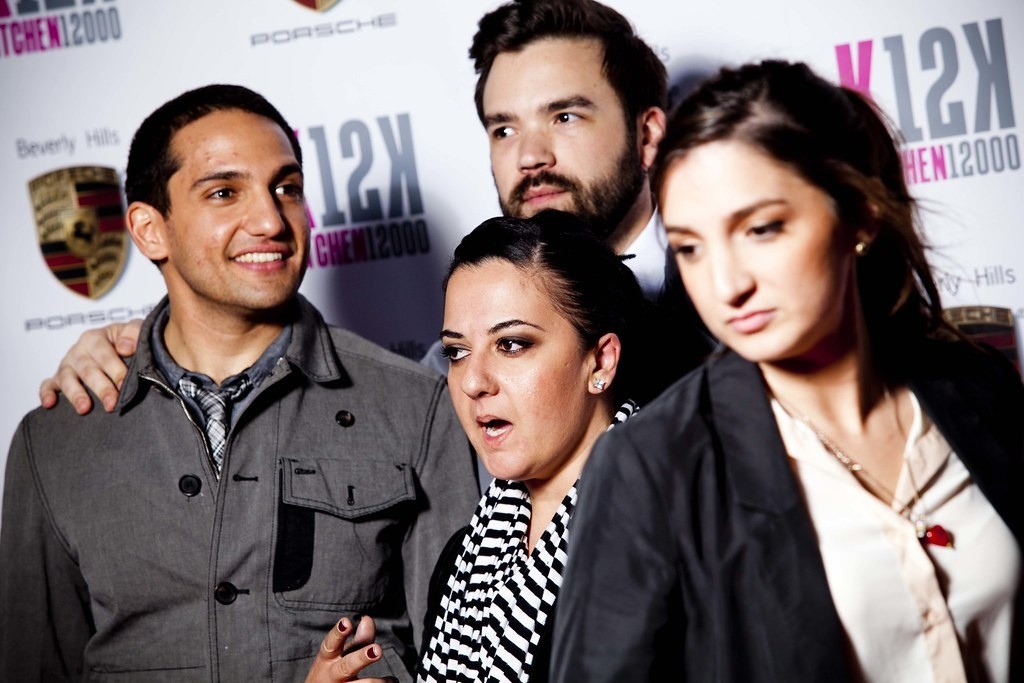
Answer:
[781,387,952,546]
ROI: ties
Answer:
[178,373,253,476]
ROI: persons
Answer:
[37,0,715,498]
[548,64,1024,683]
[314,208,658,683]
[0,83,481,683]
